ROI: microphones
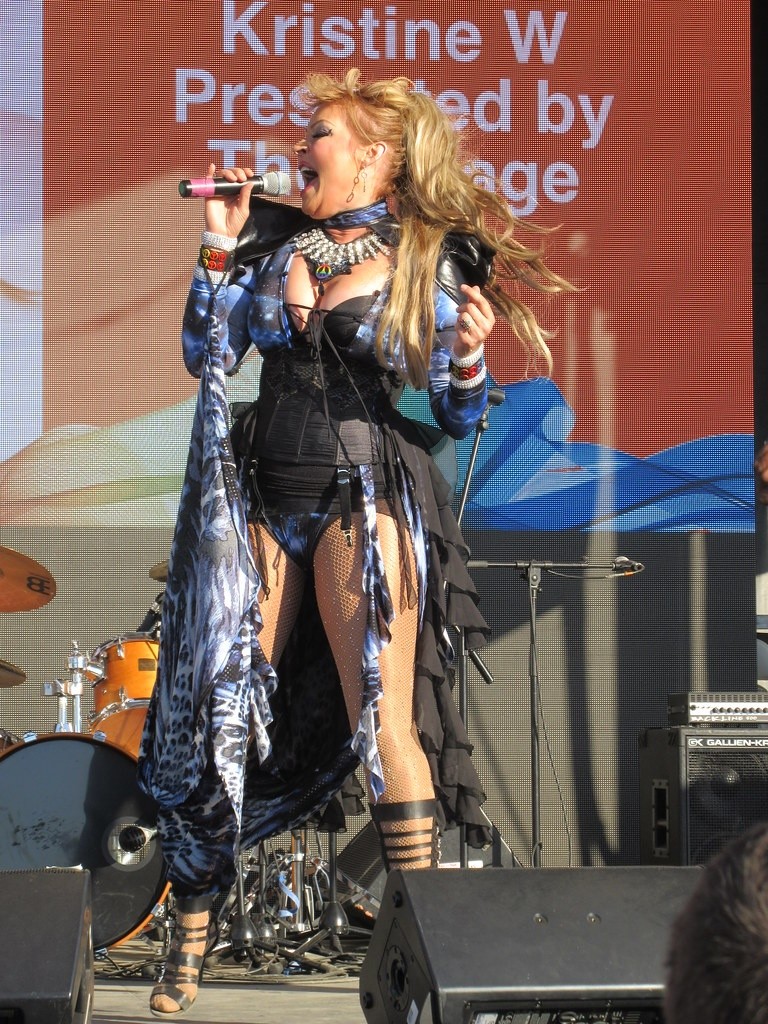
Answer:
[613,556,645,572]
[118,826,159,854]
[136,593,164,633]
[178,172,292,198]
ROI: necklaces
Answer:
[291,228,391,296]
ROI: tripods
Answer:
[144,829,375,981]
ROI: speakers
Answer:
[361,866,712,1024]
[314,804,526,927]
[635,724,768,869]
[0,868,94,1024]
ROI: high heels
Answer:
[149,894,221,1017]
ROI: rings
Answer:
[461,318,471,331]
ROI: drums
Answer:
[0,728,22,752]
[0,732,172,958]
[91,632,161,757]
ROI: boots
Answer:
[367,797,435,874]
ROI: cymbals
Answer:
[0,545,58,614]
[149,559,168,582]
[0,657,27,687]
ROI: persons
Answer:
[660,824,768,1024]
[132,65,580,1018]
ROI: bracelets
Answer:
[450,367,486,391]
[448,343,484,368]
[200,230,238,251]
[196,246,236,271]
[447,354,484,380]
[193,265,231,285]
